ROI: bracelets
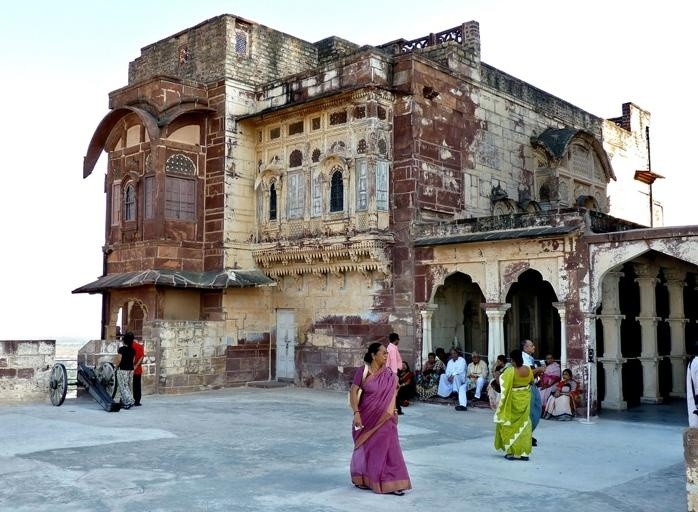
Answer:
[394,407,398,413]
[352,409,359,415]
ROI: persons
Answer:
[491,348,535,461]
[397,359,415,407]
[454,349,488,411]
[385,332,406,415]
[685,352,698,431]
[487,350,581,422]
[113,332,136,410]
[349,341,412,495]
[128,329,144,407]
[434,347,468,402]
[416,352,445,402]
[520,338,548,447]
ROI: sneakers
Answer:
[472,398,480,401]
[532,437,537,447]
[398,412,403,415]
[505,454,529,460]
[455,406,467,411]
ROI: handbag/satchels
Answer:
[491,380,501,393]
